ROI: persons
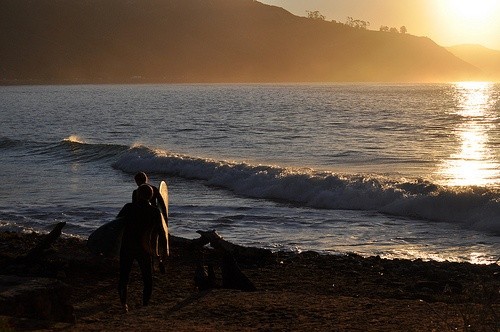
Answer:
[116,185,160,314]
[132,172,169,274]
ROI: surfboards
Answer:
[156,180,171,258]
